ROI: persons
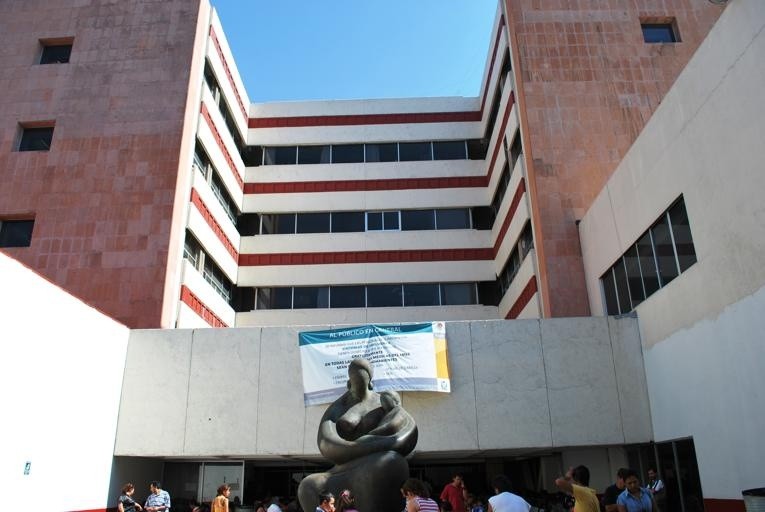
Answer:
[189,500,201,512]
[357,390,408,449]
[316,489,357,511]
[144,480,172,512]
[210,483,231,511]
[296,356,410,511]
[554,465,665,511]
[400,472,532,511]
[117,481,143,512]
